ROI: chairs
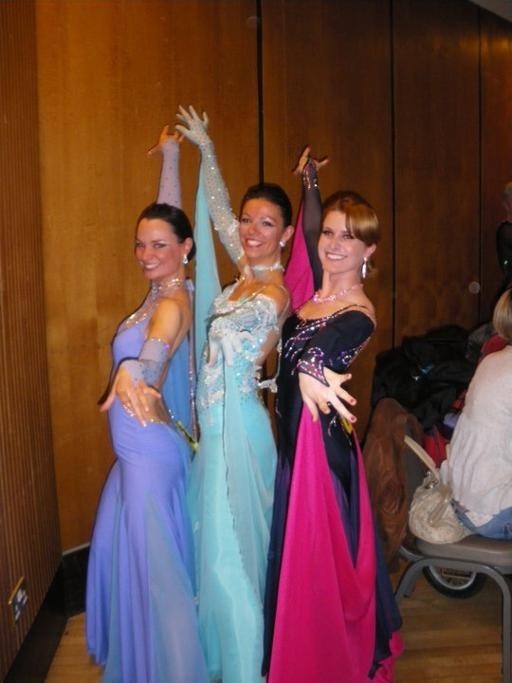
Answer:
[369,396,512,682]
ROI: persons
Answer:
[174,103,295,682]
[86,125,213,682]
[441,282,512,540]
[262,148,405,682]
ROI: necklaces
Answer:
[153,276,180,293]
[313,284,362,304]
[251,263,285,272]
